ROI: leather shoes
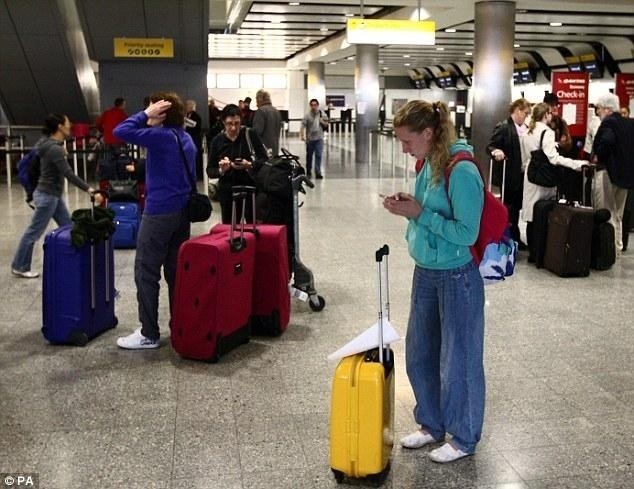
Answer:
[12,268,39,277]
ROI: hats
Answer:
[594,93,621,112]
[544,92,559,105]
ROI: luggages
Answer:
[531,165,615,278]
[42,190,118,346]
[106,173,141,249]
[171,185,290,362]
[332,245,395,486]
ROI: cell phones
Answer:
[235,158,243,162]
[379,193,395,200]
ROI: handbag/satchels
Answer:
[320,119,328,132]
[70,206,115,246]
[17,148,40,203]
[98,155,146,181]
[252,156,305,191]
[109,179,139,202]
[528,150,564,187]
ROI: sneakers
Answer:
[401,430,438,448]
[429,444,468,462]
[118,327,160,349]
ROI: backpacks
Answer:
[438,151,518,285]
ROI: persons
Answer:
[95,89,281,225]
[11,112,96,278]
[486,92,634,264]
[111,91,197,350]
[301,99,329,179]
[382,99,485,463]
